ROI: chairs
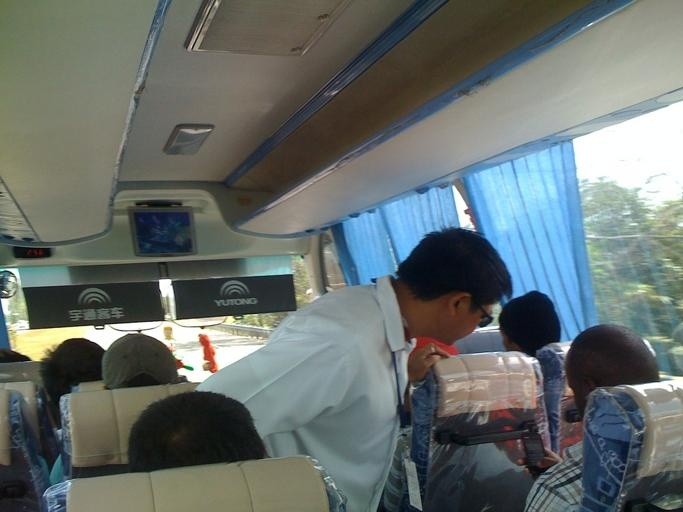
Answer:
[52,453,344,511]
[408,348,554,509]
[573,377,682,510]
[1,361,54,409]
[59,380,204,475]
[2,383,56,462]
[540,334,657,457]
[451,328,510,355]
[0,389,49,510]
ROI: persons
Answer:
[195,222,514,512]
[40,338,107,404]
[49,331,178,487]
[126,388,271,474]
[520,322,661,511]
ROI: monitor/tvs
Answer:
[126,206,197,256]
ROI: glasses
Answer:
[471,296,494,329]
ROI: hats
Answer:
[101,334,177,390]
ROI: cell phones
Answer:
[521,432,550,481]
